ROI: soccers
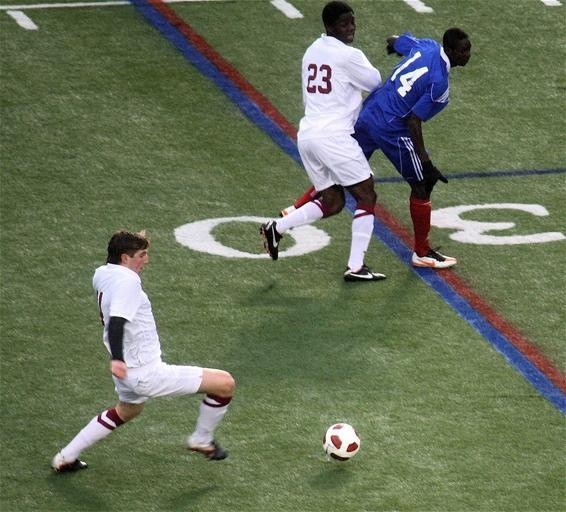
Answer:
[321,423,360,461]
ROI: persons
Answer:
[50,228,236,473]
[259,1,390,282]
[278,27,473,268]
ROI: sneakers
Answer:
[341,266,387,282]
[186,438,228,462]
[260,221,282,261]
[282,210,293,231]
[409,249,459,271]
[51,458,86,473]
